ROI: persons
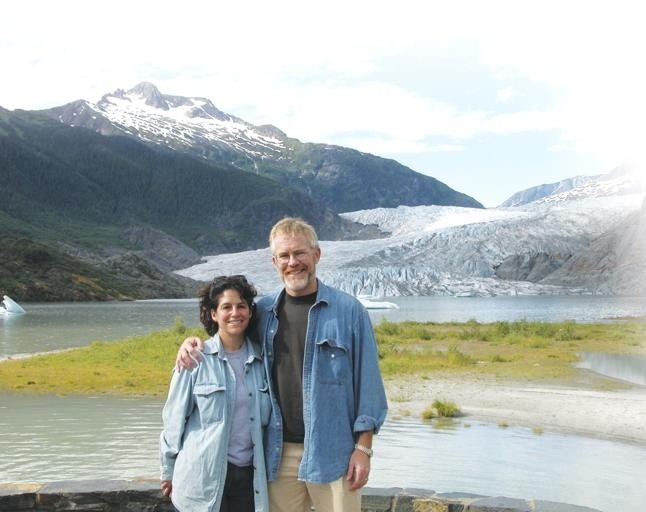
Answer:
[174,212,389,512]
[158,273,272,512]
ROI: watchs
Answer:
[354,443,374,457]
